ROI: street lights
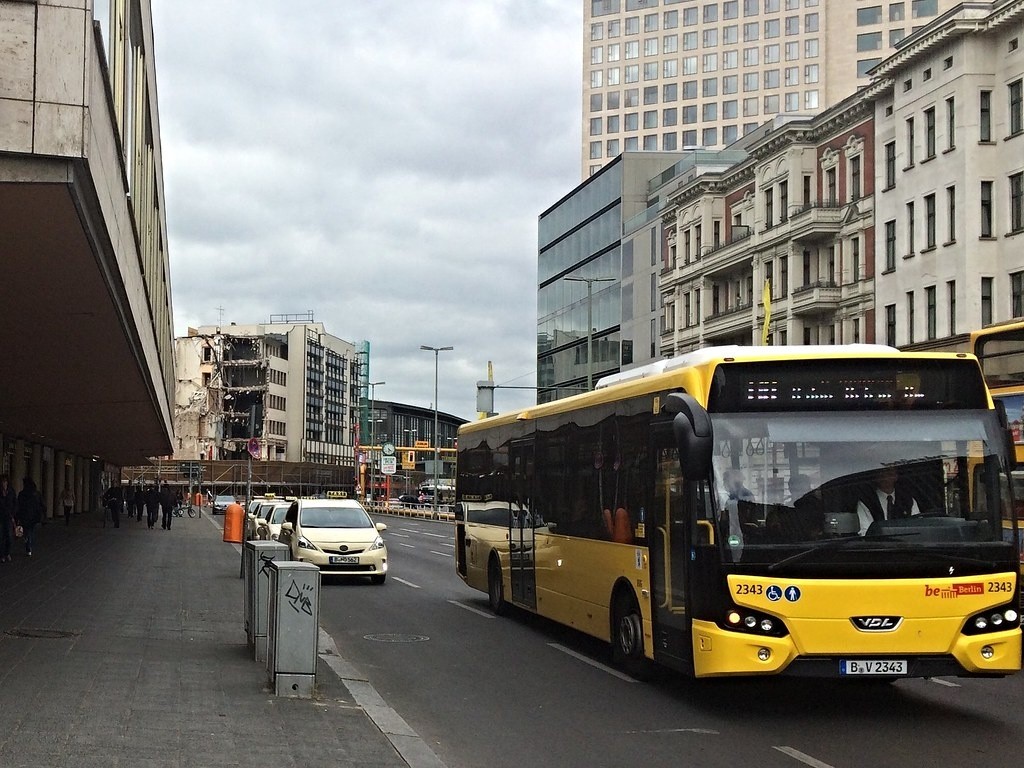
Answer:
[361,382,385,512]
[368,420,383,500]
[404,430,417,447]
[447,437,458,500]
[420,345,453,520]
[562,274,617,392]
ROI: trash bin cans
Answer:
[603,509,612,535]
[223,504,245,542]
[195,493,202,506]
[613,508,632,544]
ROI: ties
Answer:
[887,494,895,522]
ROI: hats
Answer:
[162,484,171,488]
[148,484,155,487]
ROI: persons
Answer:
[857,456,922,537]
[723,470,756,558]
[100,481,190,530]
[58,483,75,525]
[0,474,45,563]
[207,489,212,505]
[787,473,822,508]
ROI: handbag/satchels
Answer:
[15,524,24,538]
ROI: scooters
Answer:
[171,498,196,518]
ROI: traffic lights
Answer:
[408,451,415,463]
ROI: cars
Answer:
[248,500,284,529]
[247,502,292,541]
[213,495,237,515]
[278,499,389,585]
[457,502,558,571]
[257,505,290,542]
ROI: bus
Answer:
[421,486,456,505]
[967,322,1024,617]
[456,345,1023,686]
[369,474,414,501]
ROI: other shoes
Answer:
[6,555,12,562]
[113,511,171,530]
[1,556,6,562]
[25,551,32,556]
